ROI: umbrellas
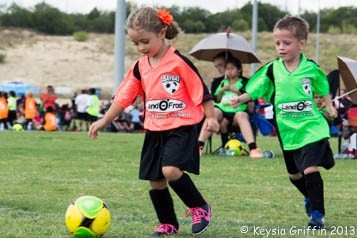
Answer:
[189,31,261,78]
[331,56,357,108]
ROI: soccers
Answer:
[65,194,111,238]
[224,139,244,156]
[12,123,23,132]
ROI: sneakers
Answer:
[305,198,310,216]
[249,148,265,158]
[151,224,181,237]
[184,204,212,235]
[308,211,324,228]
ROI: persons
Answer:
[314,91,324,107]
[0,86,143,133]
[198,51,264,159]
[87,7,220,237]
[212,59,248,115]
[339,88,348,103]
[231,16,338,229]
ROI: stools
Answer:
[206,77,258,154]
